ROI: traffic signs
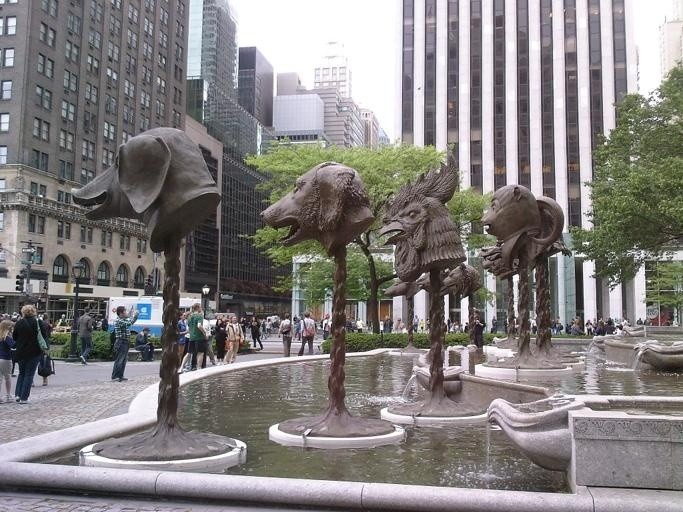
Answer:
[20,259,33,265]
[26,283,32,294]
[21,247,36,255]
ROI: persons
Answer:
[177,303,244,375]
[1,305,53,403]
[474,319,485,348]
[636,317,651,326]
[345,314,432,334]
[445,318,469,334]
[112,306,139,381]
[57,314,108,332]
[549,317,632,337]
[238,311,333,358]
[135,327,154,361]
[78,307,93,364]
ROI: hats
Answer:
[143,328,150,332]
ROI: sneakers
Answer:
[1,394,27,404]
[178,360,236,374]
[111,378,120,383]
[79,356,86,363]
[119,376,128,382]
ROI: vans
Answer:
[208,312,236,335]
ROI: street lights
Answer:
[201,283,210,319]
[63,261,85,361]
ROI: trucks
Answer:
[101,296,216,339]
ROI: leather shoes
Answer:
[42,378,49,386]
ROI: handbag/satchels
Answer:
[304,328,314,337]
[36,331,48,351]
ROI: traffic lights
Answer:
[147,274,153,288]
[143,278,148,288]
[14,273,24,292]
[0,242,2,253]
[43,280,48,290]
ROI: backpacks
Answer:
[281,327,291,334]
[38,354,55,376]
